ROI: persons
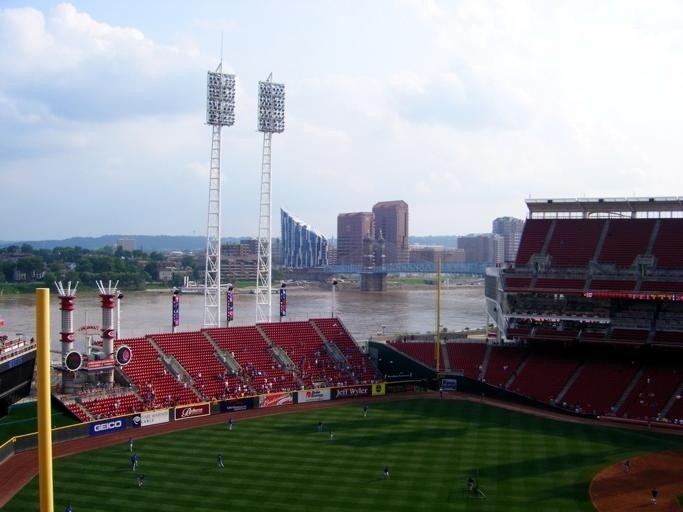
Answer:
[467,478,474,496]
[227,416,232,431]
[549,396,615,418]
[649,486,657,503]
[624,458,630,472]
[363,406,368,417]
[127,436,134,452]
[129,453,137,470]
[382,465,390,479]
[624,412,680,424]
[316,419,322,431]
[328,429,335,439]
[63,504,72,511]
[439,389,444,400]
[481,393,485,401]
[505,288,682,340]
[135,473,144,487]
[216,454,224,471]
[77,341,378,423]
